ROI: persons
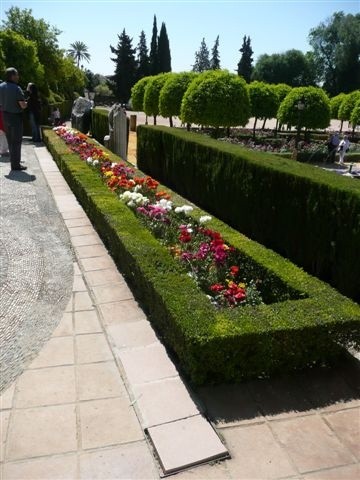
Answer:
[0,67,28,170]
[25,82,43,142]
[331,130,350,166]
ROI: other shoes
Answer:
[29,136,34,140]
[2,152,9,157]
[13,164,26,170]
[33,137,40,141]
